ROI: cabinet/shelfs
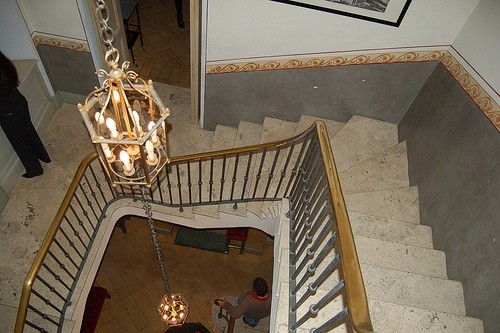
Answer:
[121,1,144,68]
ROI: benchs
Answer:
[174,228,228,254]
[80,286,112,333]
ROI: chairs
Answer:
[226,228,249,254]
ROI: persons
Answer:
[0,51,51,178]
[217,277,271,327]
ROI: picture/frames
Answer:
[270,0,412,28]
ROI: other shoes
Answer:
[21,170,43,178]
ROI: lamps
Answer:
[77,1,190,328]
